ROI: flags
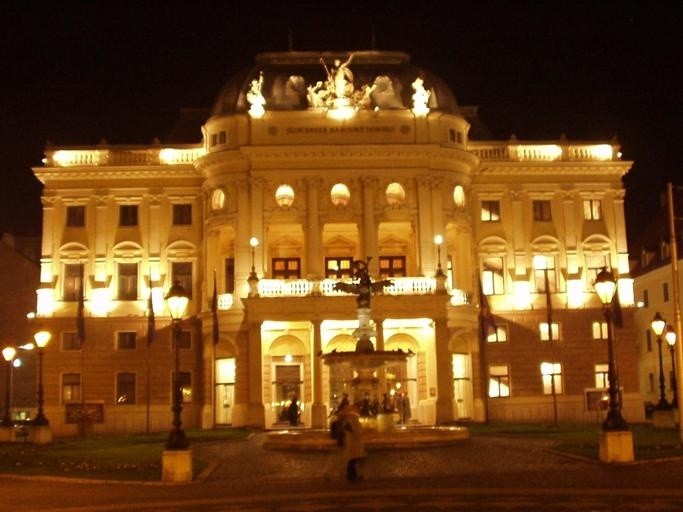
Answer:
[76,267,85,346]
[212,278,219,345]
[605,270,624,327]
[544,269,552,325]
[148,278,155,347]
[480,280,497,342]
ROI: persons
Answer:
[336,404,367,481]
[305,53,377,109]
[286,391,411,425]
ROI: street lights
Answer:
[26,323,55,446]
[159,274,192,484]
[2,343,22,440]
[648,311,679,428]
[591,266,634,464]
